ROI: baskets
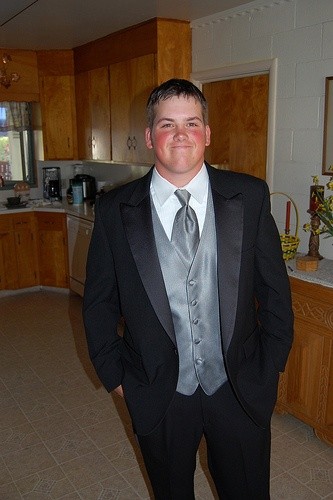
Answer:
[269,192,300,261]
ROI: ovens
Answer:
[66,214,94,296]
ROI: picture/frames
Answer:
[321,76,333,176]
[189,58,277,206]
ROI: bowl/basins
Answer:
[7,197,20,204]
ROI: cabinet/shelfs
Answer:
[274,253,333,447]
[35,212,69,289]
[30,48,77,161]
[74,35,112,164]
[0,212,40,291]
[108,18,192,166]
[0,49,39,103]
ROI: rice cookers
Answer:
[42,166,63,202]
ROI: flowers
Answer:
[302,164,333,246]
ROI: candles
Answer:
[285,201,290,231]
[310,186,324,210]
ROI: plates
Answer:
[5,203,28,209]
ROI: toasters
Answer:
[69,173,97,200]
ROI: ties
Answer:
[171,190,199,274]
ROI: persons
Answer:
[83,78,295,500]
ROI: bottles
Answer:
[71,184,83,206]
